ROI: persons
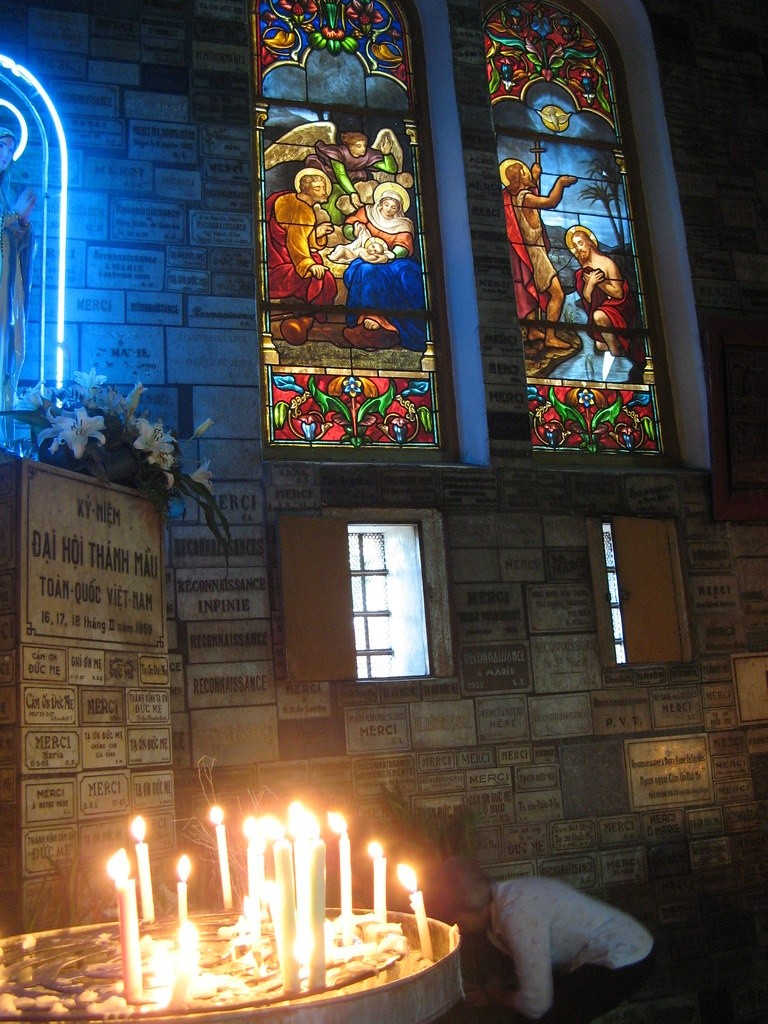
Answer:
[434,857,655,1024]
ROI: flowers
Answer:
[0,367,237,585]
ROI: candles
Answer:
[366,842,387,919]
[105,800,325,1002]
[328,809,353,912]
[397,862,434,963]
